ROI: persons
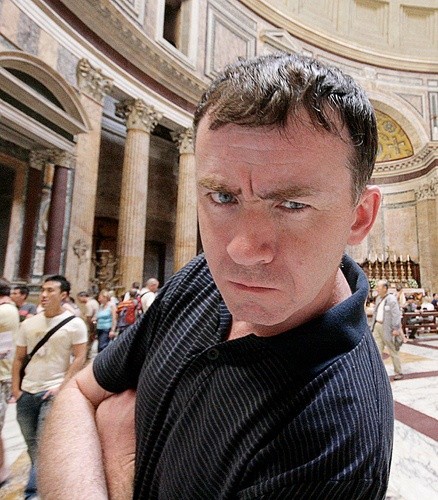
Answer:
[124,281,141,301]
[420,296,434,333]
[11,286,36,322]
[109,291,118,305]
[68,297,80,312]
[432,294,438,311]
[36,286,77,316]
[120,288,143,333]
[401,296,419,339]
[0,284,20,488]
[77,291,99,345]
[37,54,395,500]
[91,289,119,353]
[366,279,404,380]
[369,297,377,311]
[12,275,89,500]
[425,292,431,302]
[138,278,159,314]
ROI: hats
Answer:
[395,335,403,351]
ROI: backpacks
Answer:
[136,292,146,314]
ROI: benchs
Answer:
[401,310,438,337]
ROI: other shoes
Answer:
[394,374,402,379]
